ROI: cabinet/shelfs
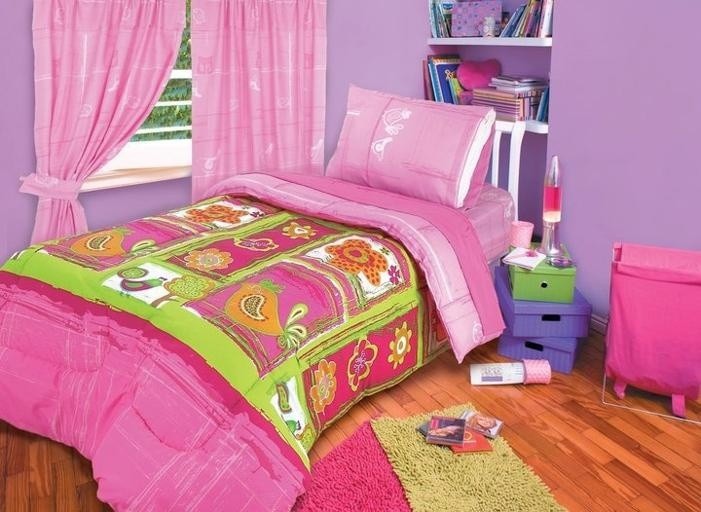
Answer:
[427,0,553,135]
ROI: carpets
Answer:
[289,404,568,512]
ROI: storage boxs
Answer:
[472,86,546,122]
[495,242,594,376]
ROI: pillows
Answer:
[325,83,496,208]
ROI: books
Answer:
[502,246,547,271]
[420,411,503,454]
[428,0,554,123]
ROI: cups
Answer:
[510,221,534,248]
[522,359,551,385]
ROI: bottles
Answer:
[483,16,495,37]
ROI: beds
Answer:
[14,116,526,512]
